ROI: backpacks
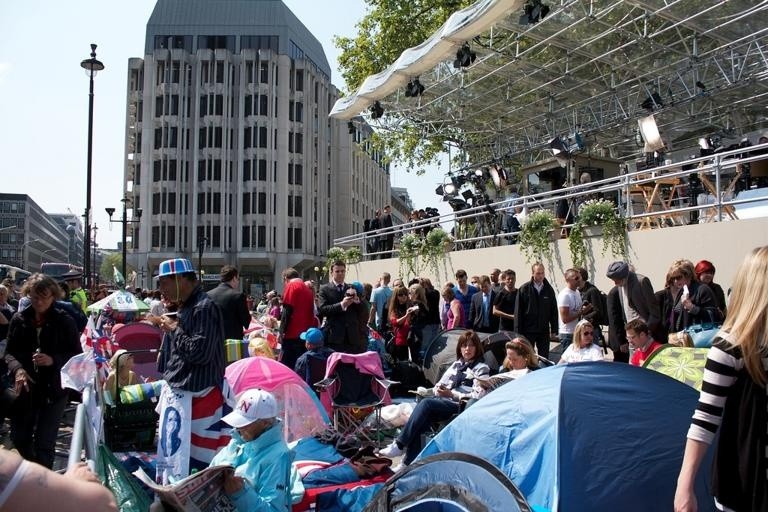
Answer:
[599,291,609,326]
[390,360,426,398]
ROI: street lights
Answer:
[103,193,143,286]
[0,225,16,231]
[79,42,105,289]
[91,221,98,288]
[19,239,39,269]
[39,248,56,264]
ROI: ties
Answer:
[338,284,343,293]
[485,294,489,322]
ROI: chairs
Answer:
[103,349,165,446]
[286,451,298,512]
[408,362,471,437]
[313,350,401,447]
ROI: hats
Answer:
[220,388,278,428]
[607,262,628,280]
[154,259,196,280]
[300,328,325,344]
[62,272,82,280]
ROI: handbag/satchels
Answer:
[1,380,32,422]
[385,332,395,353]
[669,332,693,348]
[687,317,721,347]
[370,237,378,252]
[654,318,670,344]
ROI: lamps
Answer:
[349,120,356,136]
[368,99,385,120]
[434,128,717,206]
[403,74,427,98]
[452,42,478,70]
[516,1,551,28]
[641,92,666,114]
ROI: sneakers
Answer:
[379,441,402,457]
[392,464,407,473]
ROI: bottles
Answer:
[33,348,41,374]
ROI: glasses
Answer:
[584,331,594,336]
[28,295,52,301]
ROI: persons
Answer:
[758,137,768,144]
[362,204,441,260]
[673,244,768,511]
[2,254,732,511]
[502,171,599,245]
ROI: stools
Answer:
[623,160,742,231]
